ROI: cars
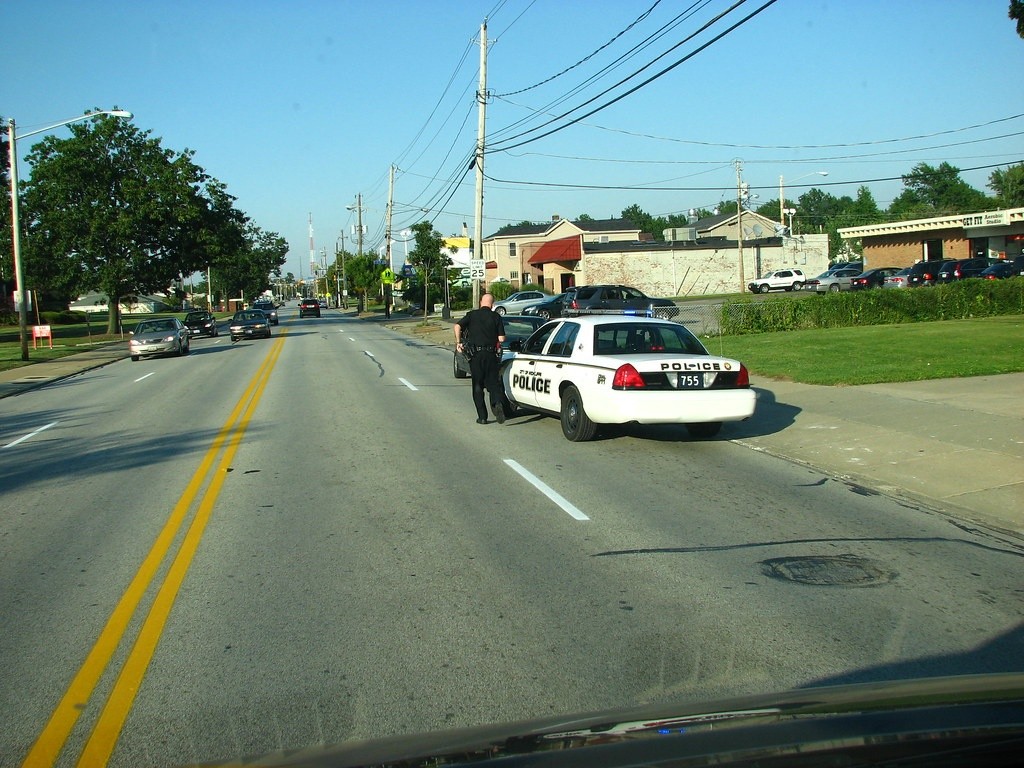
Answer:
[491,290,563,316]
[521,292,566,325]
[1014,254,1024,272]
[499,310,757,442]
[228,309,272,342]
[129,317,191,362]
[831,264,863,274]
[807,267,863,295]
[977,262,1022,280]
[183,311,219,338]
[850,267,902,291]
[884,266,911,288]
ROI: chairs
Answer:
[165,322,174,329]
[625,334,645,350]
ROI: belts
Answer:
[468,346,497,352]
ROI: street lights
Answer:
[736,193,760,295]
[778,172,827,228]
[344,205,367,314]
[782,208,796,238]
[385,208,429,318]
[8,111,133,359]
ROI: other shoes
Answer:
[477,417,488,423]
[495,400,505,424]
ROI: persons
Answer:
[774,273,779,279]
[239,315,246,321]
[454,294,505,424]
[383,292,392,318]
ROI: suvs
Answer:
[254,300,279,325]
[298,298,321,319]
[561,284,680,320]
[937,258,1008,284]
[748,268,807,294]
[906,258,957,288]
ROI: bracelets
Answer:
[457,341,462,344]
